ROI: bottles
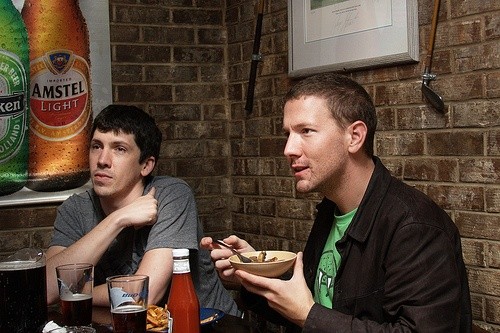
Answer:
[167,249,200,333]
[20,0,91,192]
[0,0,29,196]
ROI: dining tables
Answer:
[48,301,251,333]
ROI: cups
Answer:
[106,274,149,333]
[56,262,94,327]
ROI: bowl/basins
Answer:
[228,251,297,278]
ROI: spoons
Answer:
[210,236,253,263]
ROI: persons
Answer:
[200,74,472,333]
[46,105,244,318]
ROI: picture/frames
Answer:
[287,0,420,79]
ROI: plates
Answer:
[159,304,225,332]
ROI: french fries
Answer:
[145,304,168,331]
